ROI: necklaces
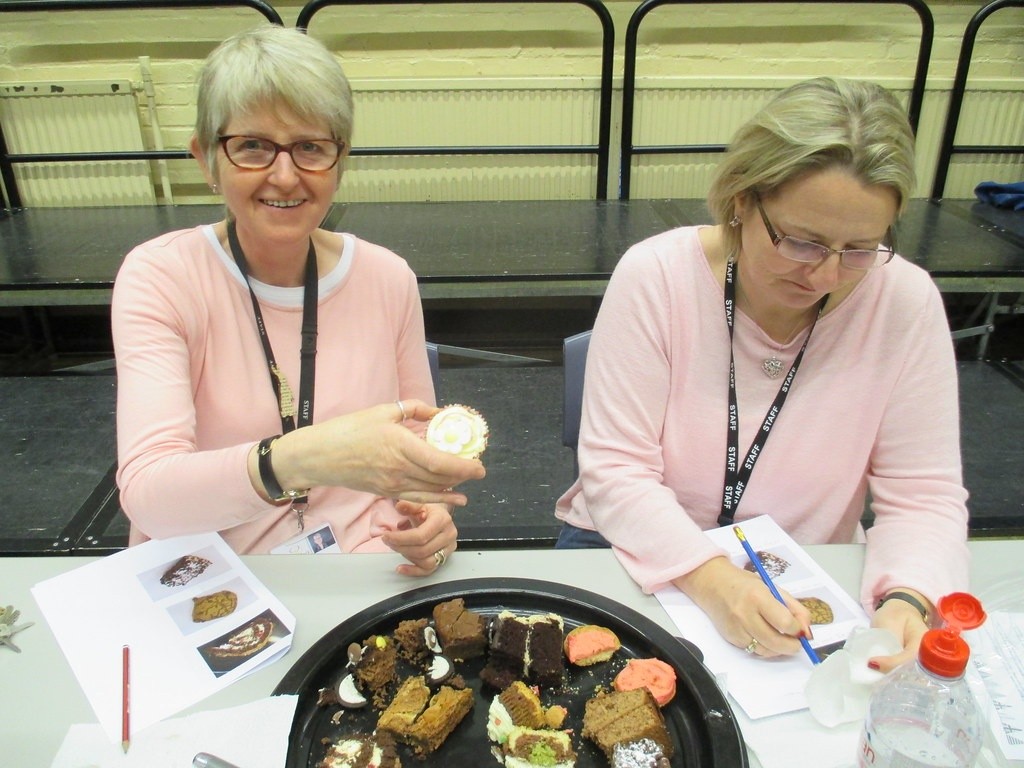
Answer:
[736,280,807,381]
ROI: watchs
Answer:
[877,592,930,624]
[258,435,311,503]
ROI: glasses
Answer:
[215,131,347,176]
[754,194,896,269]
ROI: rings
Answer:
[434,549,446,567]
[747,639,758,653]
[397,400,407,422]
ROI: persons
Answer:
[107,27,489,577]
[311,532,329,553]
[551,78,973,674]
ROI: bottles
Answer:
[858,592,987,767]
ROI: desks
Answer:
[0,537,1024,768]
[0,198,1024,373]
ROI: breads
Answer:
[192,591,237,623]
[159,556,211,588]
[744,551,791,583]
[320,597,678,768]
[204,618,274,657]
[797,597,834,626]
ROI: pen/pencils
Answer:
[733,525,822,668]
[122,645,131,755]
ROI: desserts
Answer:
[424,403,489,459]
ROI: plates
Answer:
[271,576,750,768]
